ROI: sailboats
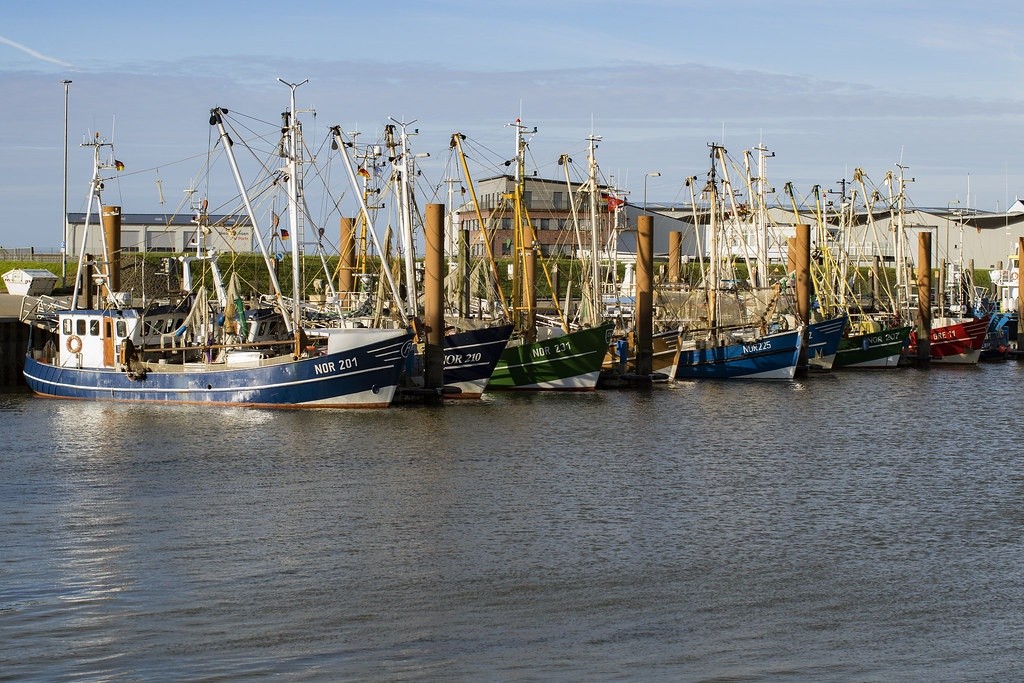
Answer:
[18,78,1021,410]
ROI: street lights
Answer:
[945,200,960,283]
[644,173,661,216]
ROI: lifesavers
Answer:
[1011,272,1018,280]
[66,335,83,353]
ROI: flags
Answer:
[281,229,290,240]
[601,193,609,201]
[358,166,370,177]
[116,161,124,170]
